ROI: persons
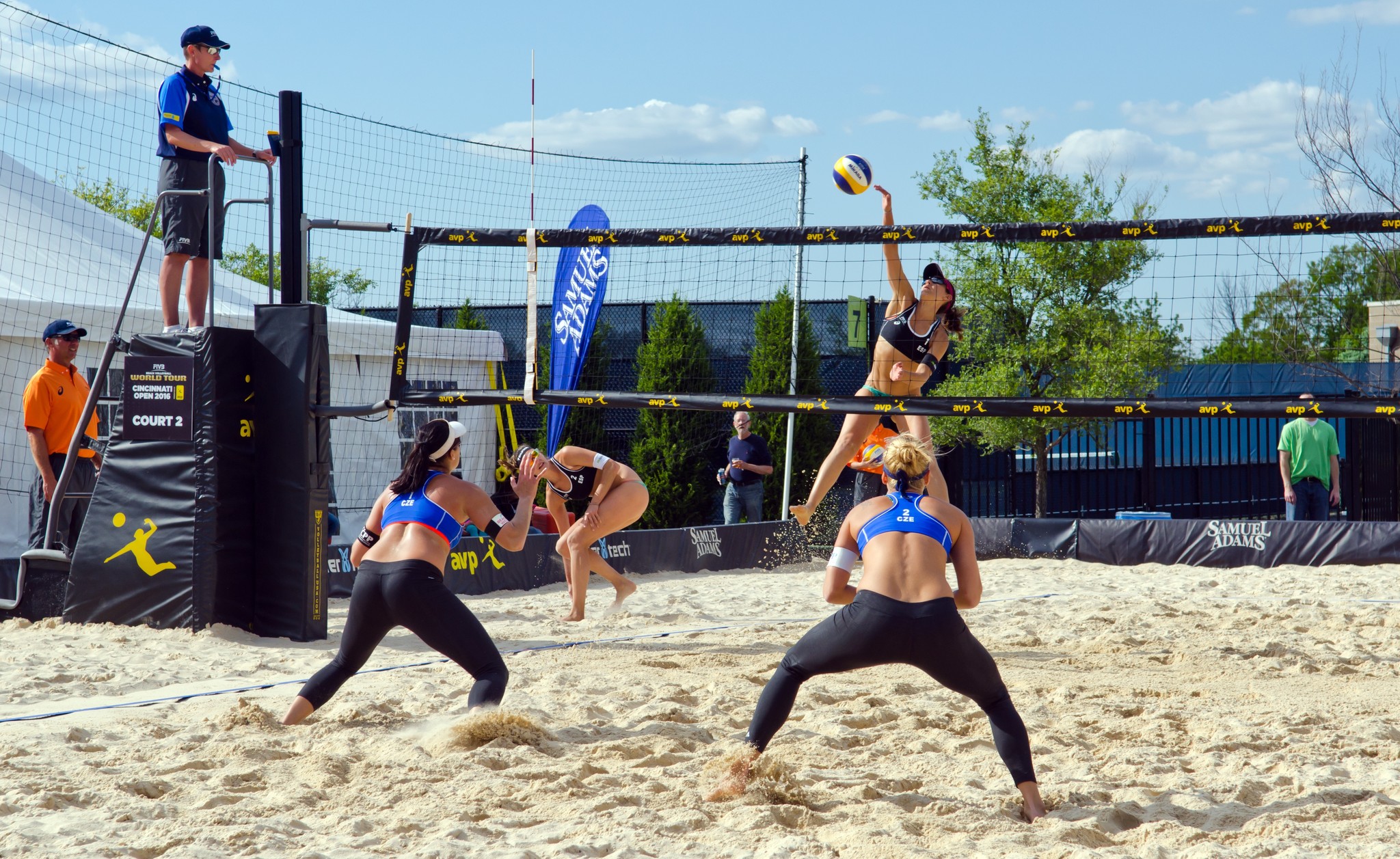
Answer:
[744,433,1045,822]
[498,444,649,622]
[846,422,899,508]
[1277,392,1340,521]
[716,411,773,524]
[789,185,963,526]
[288,418,546,725]
[24,320,101,552]
[156,25,276,335]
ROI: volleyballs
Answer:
[833,154,874,196]
[863,444,885,467]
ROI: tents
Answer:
[1,151,507,559]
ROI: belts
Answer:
[1304,477,1316,482]
[731,480,756,487]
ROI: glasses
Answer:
[50,334,80,343]
[922,277,945,286]
[195,44,221,55]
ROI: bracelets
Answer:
[253,150,261,158]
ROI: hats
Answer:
[923,263,953,310]
[42,319,87,342]
[427,419,466,462]
[180,25,230,50]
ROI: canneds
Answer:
[718,468,726,484]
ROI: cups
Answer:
[732,458,740,468]
[718,468,727,485]
